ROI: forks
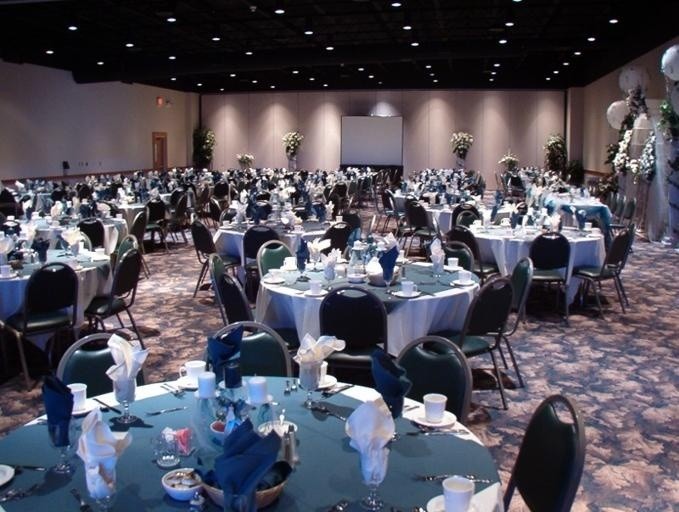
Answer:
[0,463,47,503]
[70,487,94,512]
[411,473,475,483]
[99,405,109,412]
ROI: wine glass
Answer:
[360,448,388,510]
[45,425,75,475]
[299,361,319,410]
[113,375,140,424]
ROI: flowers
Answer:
[497,152,519,170]
[449,131,474,156]
[236,153,256,166]
[281,128,304,160]
[603,83,656,182]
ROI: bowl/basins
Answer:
[210,420,226,442]
[202,460,291,511]
[162,469,206,501]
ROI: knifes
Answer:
[92,398,121,414]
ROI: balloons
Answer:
[661,44,679,81]
[618,65,652,95]
[606,99,635,131]
[671,84,679,117]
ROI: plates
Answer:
[176,376,198,390]
[411,409,458,429]
[192,389,221,400]
[71,399,99,415]
[257,419,299,437]
[298,374,338,390]
[219,378,246,391]
[426,495,476,512]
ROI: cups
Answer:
[422,392,447,423]
[261,237,478,302]
[88,455,117,509]
[149,431,181,467]
[198,372,217,398]
[443,477,474,511]
[225,361,243,388]
[0,265,17,279]
[66,383,87,410]
[246,374,268,404]
[321,359,331,384]
[219,211,343,235]
[67,240,109,271]
[22,210,125,232]
[177,360,207,387]
[470,215,600,239]
